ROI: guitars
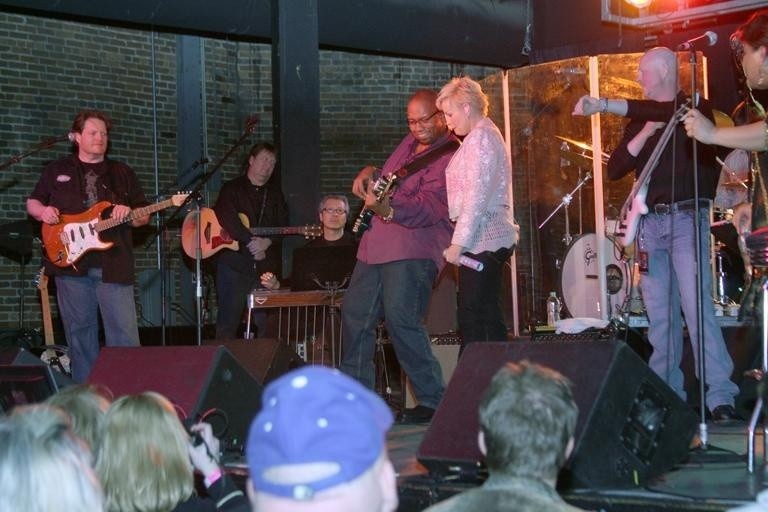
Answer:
[619,92,699,247]
[182,208,323,260]
[32,267,72,393]
[42,191,193,268]
[353,168,397,232]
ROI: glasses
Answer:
[321,206,347,214]
[406,111,437,127]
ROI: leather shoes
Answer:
[711,405,738,425]
[398,405,434,424]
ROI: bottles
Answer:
[546,290,561,324]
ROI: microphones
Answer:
[678,31,717,51]
[246,115,260,126]
[49,133,75,142]
[581,170,592,183]
[191,156,213,166]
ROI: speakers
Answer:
[202,338,304,387]
[0,347,77,420]
[416,341,702,493]
[86,346,264,452]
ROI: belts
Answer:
[649,201,706,216]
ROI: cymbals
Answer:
[555,136,611,158]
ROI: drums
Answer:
[559,233,632,322]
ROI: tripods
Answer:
[688,51,743,458]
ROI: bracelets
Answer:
[381,205,395,224]
[602,96,609,115]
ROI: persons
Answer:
[260,193,362,342]
[570,45,740,426]
[211,144,292,340]
[339,89,458,426]
[1,386,254,512]
[435,78,521,362]
[682,9,767,330]
[26,109,153,385]
[420,362,589,512]
